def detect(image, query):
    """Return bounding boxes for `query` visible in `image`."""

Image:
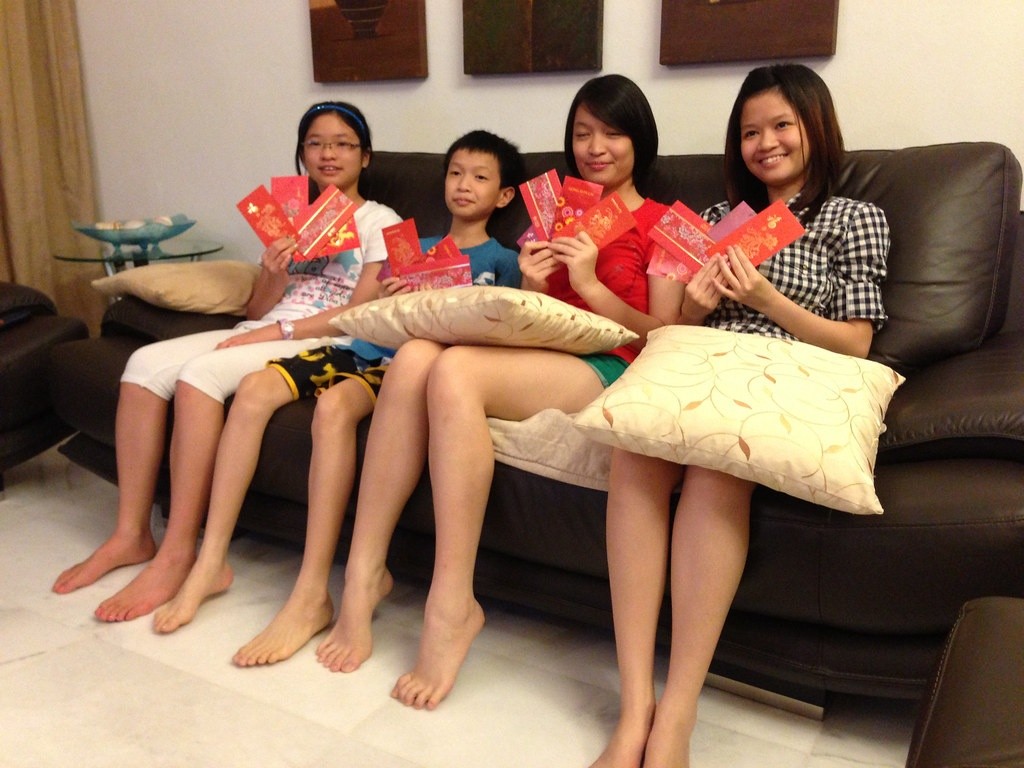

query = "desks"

[51,236,223,302]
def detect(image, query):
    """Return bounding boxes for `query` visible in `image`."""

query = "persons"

[48,100,404,624]
[150,128,523,668]
[590,62,893,767]
[317,76,676,710]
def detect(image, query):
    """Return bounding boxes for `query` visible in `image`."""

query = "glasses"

[300,139,362,154]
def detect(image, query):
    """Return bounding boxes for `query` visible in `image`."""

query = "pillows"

[90,258,265,316]
[328,285,641,354]
[572,324,906,516]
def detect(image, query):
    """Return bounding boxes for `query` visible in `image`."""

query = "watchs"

[277,318,294,341]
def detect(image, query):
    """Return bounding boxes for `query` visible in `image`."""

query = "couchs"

[0,281,90,491]
[903,596,1024,768]
[44,144,1024,704]
